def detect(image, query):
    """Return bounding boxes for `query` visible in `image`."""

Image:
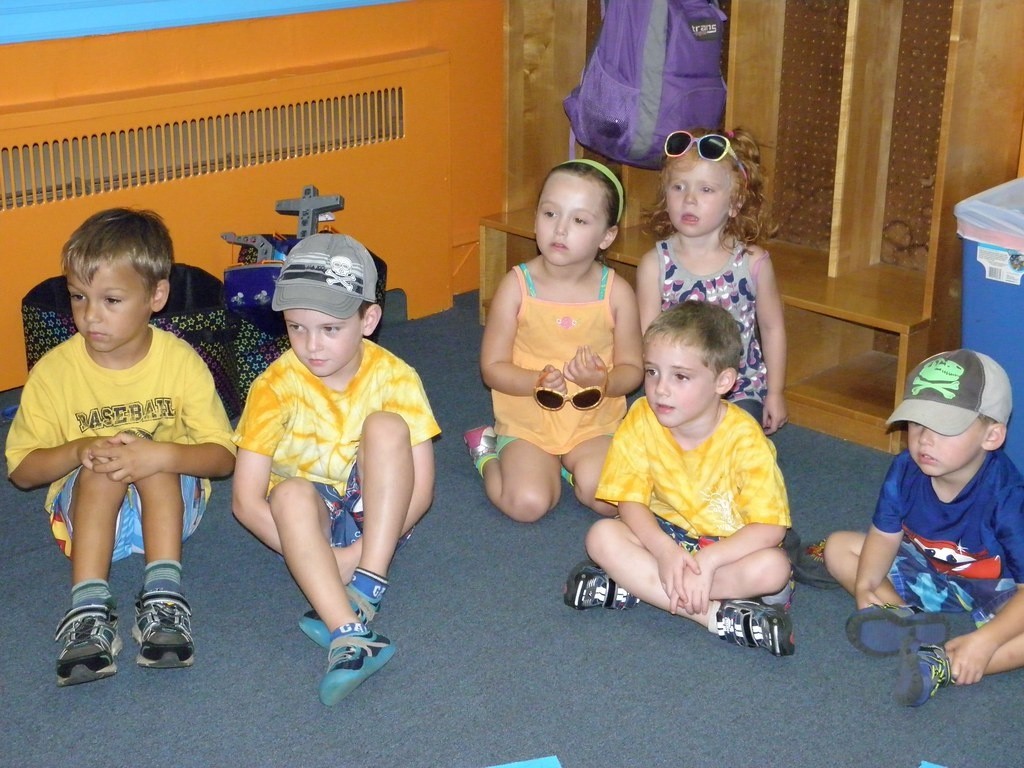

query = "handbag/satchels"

[22,261,240,422]
[222,248,387,414]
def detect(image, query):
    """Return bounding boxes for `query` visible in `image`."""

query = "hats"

[884,348,1013,438]
[270,233,376,320]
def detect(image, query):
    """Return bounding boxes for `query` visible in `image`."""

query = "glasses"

[664,126,749,190]
[533,370,609,411]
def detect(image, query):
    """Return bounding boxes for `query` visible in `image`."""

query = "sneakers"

[320,625,398,703]
[131,590,193,669]
[715,595,795,658]
[298,586,380,649]
[894,641,953,707]
[52,601,121,686]
[463,424,497,459]
[562,559,640,610]
[844,606,951,655]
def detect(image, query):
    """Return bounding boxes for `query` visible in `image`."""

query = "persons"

[4,208,240,686]
[565,300,794,656]
[636,128,790,437]
[463,159,644,522]
[824,349,1023,707]
[233,231,441,708]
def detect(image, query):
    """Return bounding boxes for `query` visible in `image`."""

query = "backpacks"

[562,2,730,171]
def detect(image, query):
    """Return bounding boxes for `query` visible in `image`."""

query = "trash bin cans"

[952,179,1024,477]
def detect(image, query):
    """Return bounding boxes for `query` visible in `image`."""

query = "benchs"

[478,205,930,456]
